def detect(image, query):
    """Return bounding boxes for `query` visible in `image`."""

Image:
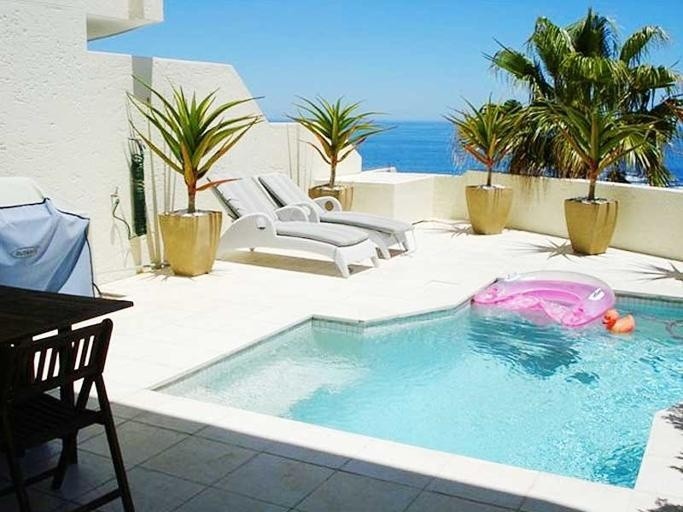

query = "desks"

[1,283,135,465]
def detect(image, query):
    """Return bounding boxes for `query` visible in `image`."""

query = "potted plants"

[123,70,266,277]
[483,5,683,257]
[440,89,522,235]
[285,90,356,210]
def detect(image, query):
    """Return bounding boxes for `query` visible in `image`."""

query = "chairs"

[204,171,380,276]
[256,170,414,259]
[1,319,138,510]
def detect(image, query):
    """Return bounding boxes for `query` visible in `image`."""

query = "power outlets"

[110,194,123,207]
